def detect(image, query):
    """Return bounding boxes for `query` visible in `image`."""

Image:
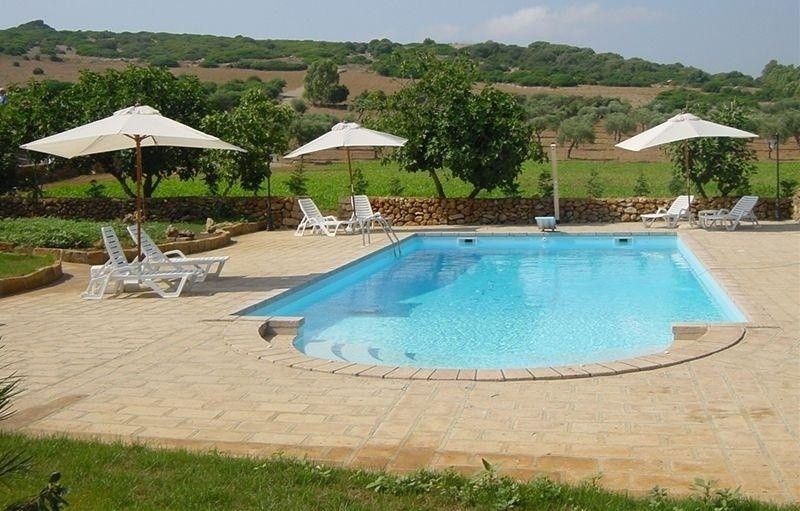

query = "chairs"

[87,222,230,298]
[700,195,760,231]
[639,194,695,228]
[347,193,381,234]
[293,197,354,237]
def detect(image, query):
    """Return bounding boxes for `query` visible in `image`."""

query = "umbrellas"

[281,118,413,227]
[15,102,253,282]
[614,112,761,225]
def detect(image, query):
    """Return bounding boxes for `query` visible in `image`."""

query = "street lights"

[769,132,782,222]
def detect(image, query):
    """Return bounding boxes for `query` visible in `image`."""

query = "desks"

[698,209,719,228]
